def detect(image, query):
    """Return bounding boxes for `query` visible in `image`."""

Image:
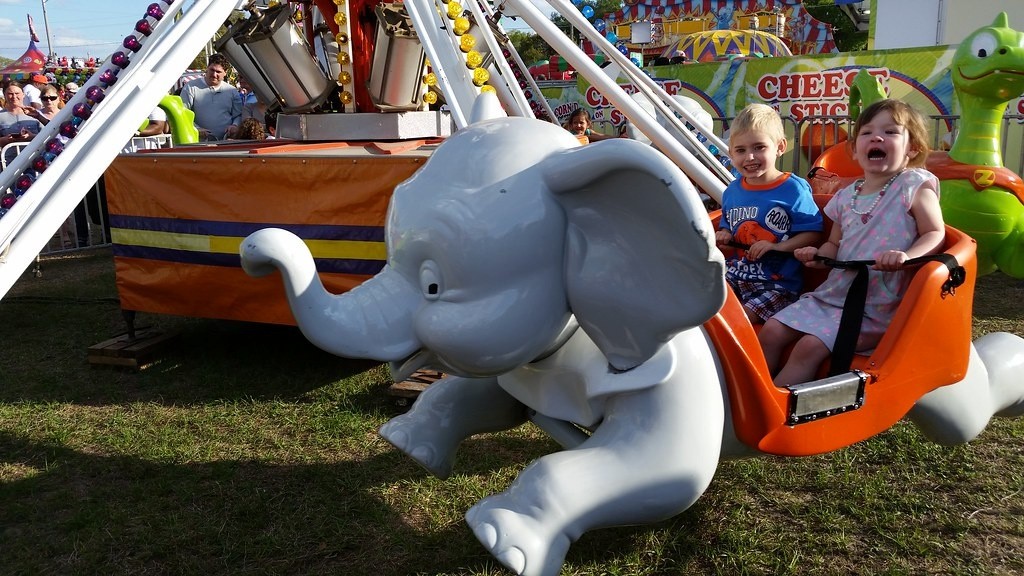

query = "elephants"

[235,113,1024,576]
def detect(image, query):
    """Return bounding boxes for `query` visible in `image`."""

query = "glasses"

[40,96,58,101]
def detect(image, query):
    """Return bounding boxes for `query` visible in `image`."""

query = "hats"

[66,82,79,92]
[33,75,47,83]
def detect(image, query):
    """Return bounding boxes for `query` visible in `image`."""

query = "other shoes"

[70,235,89,249]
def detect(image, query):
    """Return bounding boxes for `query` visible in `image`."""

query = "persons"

[757,98,945,388]
[600,55,612,68]
[37,84,72,248]
[0,79,44,174]
[0,73,184,248]
[715,103,825,323]
[180,54,243,142]
[568,107,628,143]
[235,88,283,139]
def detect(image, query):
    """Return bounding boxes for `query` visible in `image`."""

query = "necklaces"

[850,168,908,224]
